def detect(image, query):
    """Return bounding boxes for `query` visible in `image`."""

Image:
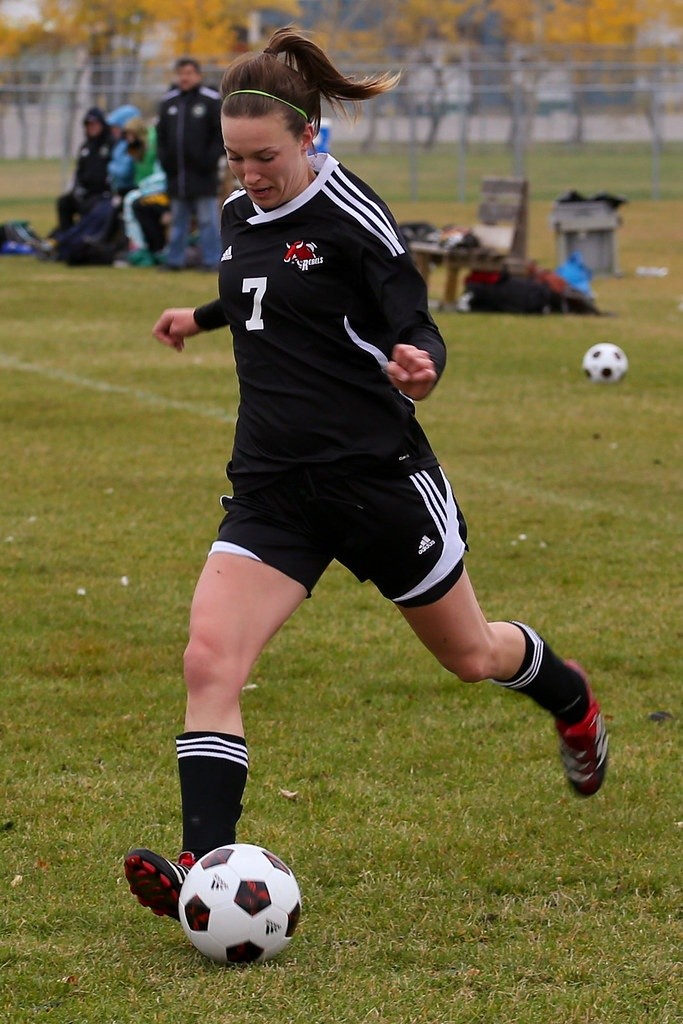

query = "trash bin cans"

[552,201,623,281]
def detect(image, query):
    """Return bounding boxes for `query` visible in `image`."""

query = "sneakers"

[552,658,606,798]
[122,845,196,921]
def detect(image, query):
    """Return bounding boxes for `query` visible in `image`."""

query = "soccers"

[179,844,301,965]
[582,342,628,384]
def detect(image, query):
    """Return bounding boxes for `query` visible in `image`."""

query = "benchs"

[407,234,511,315]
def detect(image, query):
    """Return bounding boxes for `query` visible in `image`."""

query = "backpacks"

[67,191,124,266]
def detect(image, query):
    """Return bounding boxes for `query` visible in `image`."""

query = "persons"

[118,136,161,187]
[37,104,141,259]
[152,56,228,272]
[47,105,116,246]
[121,27,614,931]
[128,192,172,259]
[84,114,151,255]
